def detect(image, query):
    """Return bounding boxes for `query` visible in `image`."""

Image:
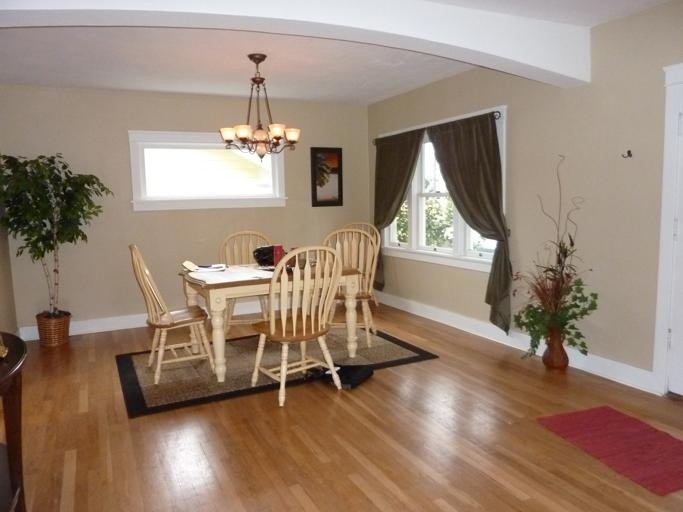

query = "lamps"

[220,54,301,161]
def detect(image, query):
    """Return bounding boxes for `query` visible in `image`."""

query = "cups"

[274,244,283,267]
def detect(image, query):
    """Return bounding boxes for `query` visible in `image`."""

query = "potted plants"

[0,152,114,347]
[509,156,598,370]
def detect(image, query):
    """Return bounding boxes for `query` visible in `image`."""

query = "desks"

[0,332,29,511]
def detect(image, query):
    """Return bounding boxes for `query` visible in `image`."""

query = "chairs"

[320,229,378,358]
[129,244,216,384]
[251,245,343,407]
[219,230,272,335]
[343,222,381,307]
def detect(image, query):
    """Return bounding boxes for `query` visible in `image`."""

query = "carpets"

[115,320,440,418]
[537,404,683,497]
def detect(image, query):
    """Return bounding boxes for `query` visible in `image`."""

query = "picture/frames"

[311,148,343,207]
